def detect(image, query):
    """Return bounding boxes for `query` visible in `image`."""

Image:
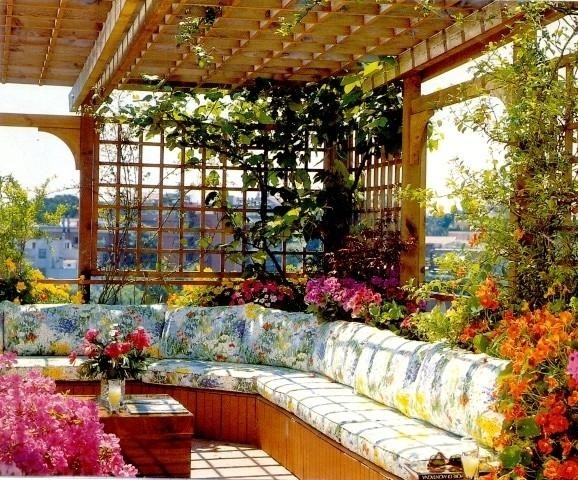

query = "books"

[122,398,190,415]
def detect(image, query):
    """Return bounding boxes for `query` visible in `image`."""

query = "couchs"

[0,298,518,479]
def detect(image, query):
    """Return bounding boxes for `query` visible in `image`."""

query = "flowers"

[64,324,153,383]
[0,353,140,479]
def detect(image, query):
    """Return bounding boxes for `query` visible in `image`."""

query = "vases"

[98,375,126,410]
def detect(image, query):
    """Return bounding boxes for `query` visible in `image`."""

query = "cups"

[107,378,122,412]
